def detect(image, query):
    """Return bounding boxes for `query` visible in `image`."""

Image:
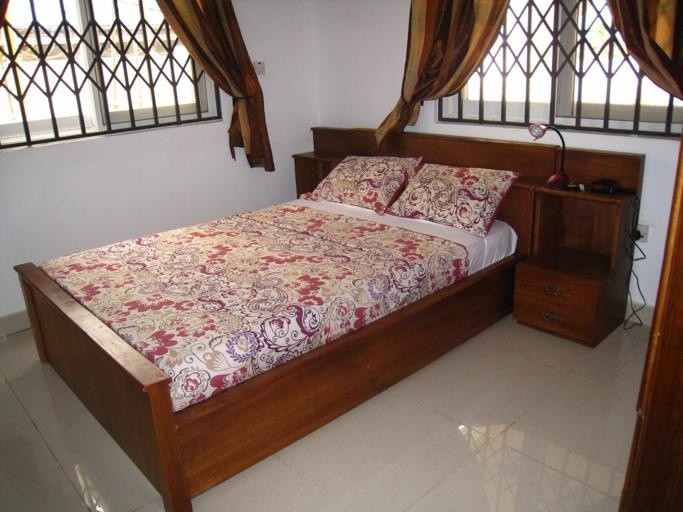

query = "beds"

[13,128,560,512]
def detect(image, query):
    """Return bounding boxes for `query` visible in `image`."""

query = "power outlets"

[636,224,649,243]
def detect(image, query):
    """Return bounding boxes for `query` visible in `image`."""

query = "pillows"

[302,156,423,216]
[384,162,524,238]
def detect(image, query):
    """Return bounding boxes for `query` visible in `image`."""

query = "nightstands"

[513,147,646,348]
[291,126,367,199]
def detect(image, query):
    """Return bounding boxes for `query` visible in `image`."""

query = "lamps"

[528,123,570,189]
